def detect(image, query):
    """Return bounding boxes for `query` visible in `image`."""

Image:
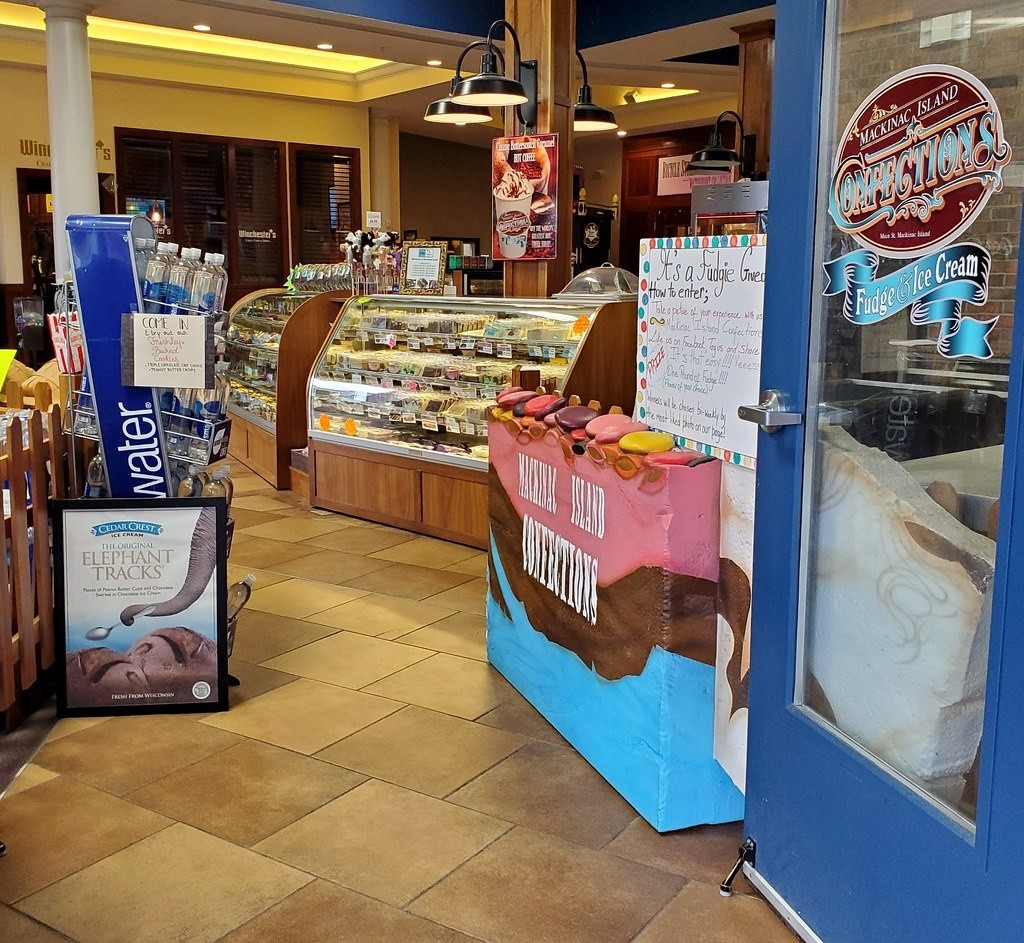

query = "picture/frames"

[402,229,417,241]
[398,240,448,295]
[46,493,229,717]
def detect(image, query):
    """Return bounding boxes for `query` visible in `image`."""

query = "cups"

[494,183,534,259]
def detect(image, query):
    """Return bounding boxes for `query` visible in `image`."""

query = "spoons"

[84,605,156,640]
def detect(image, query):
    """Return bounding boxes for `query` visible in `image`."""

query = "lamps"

[422,40,506,125]
[690,111,759,177]
[573,47,618,131]
[623,87,641,104]
[684,162,732,176]
[449,20,541,138]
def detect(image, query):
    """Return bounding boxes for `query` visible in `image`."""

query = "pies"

[495,138,550,192]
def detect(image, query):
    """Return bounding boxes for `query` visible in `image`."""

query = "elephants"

[119,507,217,628]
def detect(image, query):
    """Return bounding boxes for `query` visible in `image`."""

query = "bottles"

[73,238,233,523]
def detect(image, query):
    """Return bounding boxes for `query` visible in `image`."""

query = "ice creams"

[494,169,533,200]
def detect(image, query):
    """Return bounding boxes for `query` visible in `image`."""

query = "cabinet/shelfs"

[223,287,348,492]
[60,216,243,688]
[308,292,635,552]
